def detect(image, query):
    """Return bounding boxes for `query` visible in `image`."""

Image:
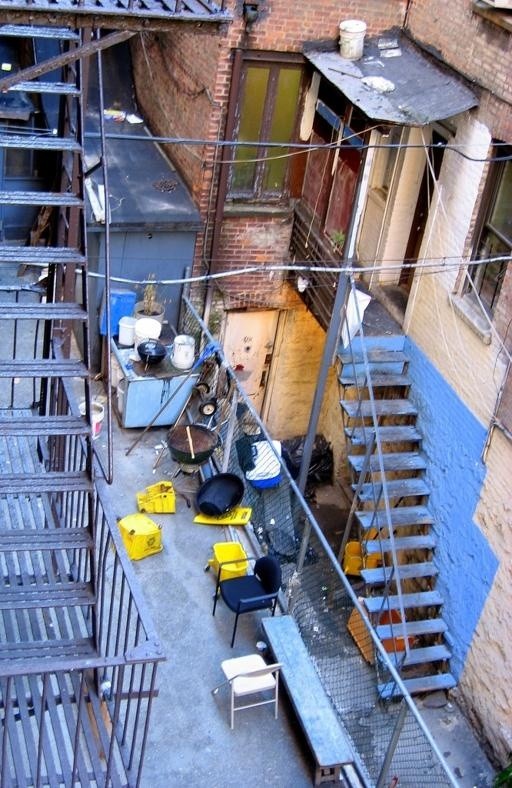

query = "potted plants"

[134,272,165,326]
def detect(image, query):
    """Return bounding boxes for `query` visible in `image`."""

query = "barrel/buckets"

[134,302,166,324]
[172,335,195,369]
[118,316,137,346]
[134,318,162,352]
[344,541,366,576]
[79,402,104,440]
[244,438,283,489]
[339,20,366,59]
[98,287,137,338]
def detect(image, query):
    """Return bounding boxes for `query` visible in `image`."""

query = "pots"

[137,339,166,366]
[196,473,245,516]
[199,400,218,416]
[166,425,219,464]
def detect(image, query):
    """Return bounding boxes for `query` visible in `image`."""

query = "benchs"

[253,614,354,787]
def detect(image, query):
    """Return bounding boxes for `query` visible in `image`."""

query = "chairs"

[212,555,283,730]
